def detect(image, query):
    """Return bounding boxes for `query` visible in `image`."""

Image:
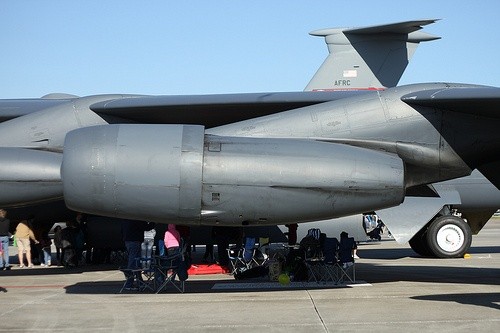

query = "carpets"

[168,263,230,275]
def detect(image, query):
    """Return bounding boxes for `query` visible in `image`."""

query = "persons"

[164,223,180,279]
[0,209,12,270]
[53,213,88,266]
[284,224,357,280]
[121,221,156,290]
[14,222,40,268]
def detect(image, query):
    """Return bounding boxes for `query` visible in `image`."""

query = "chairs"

[118,240,187,294]
[226,237,357,286]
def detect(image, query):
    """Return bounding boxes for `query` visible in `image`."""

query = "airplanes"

[0,18,499,259]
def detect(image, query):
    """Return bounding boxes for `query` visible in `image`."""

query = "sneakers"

[28,264,34,267]
[20,264,27,268]
